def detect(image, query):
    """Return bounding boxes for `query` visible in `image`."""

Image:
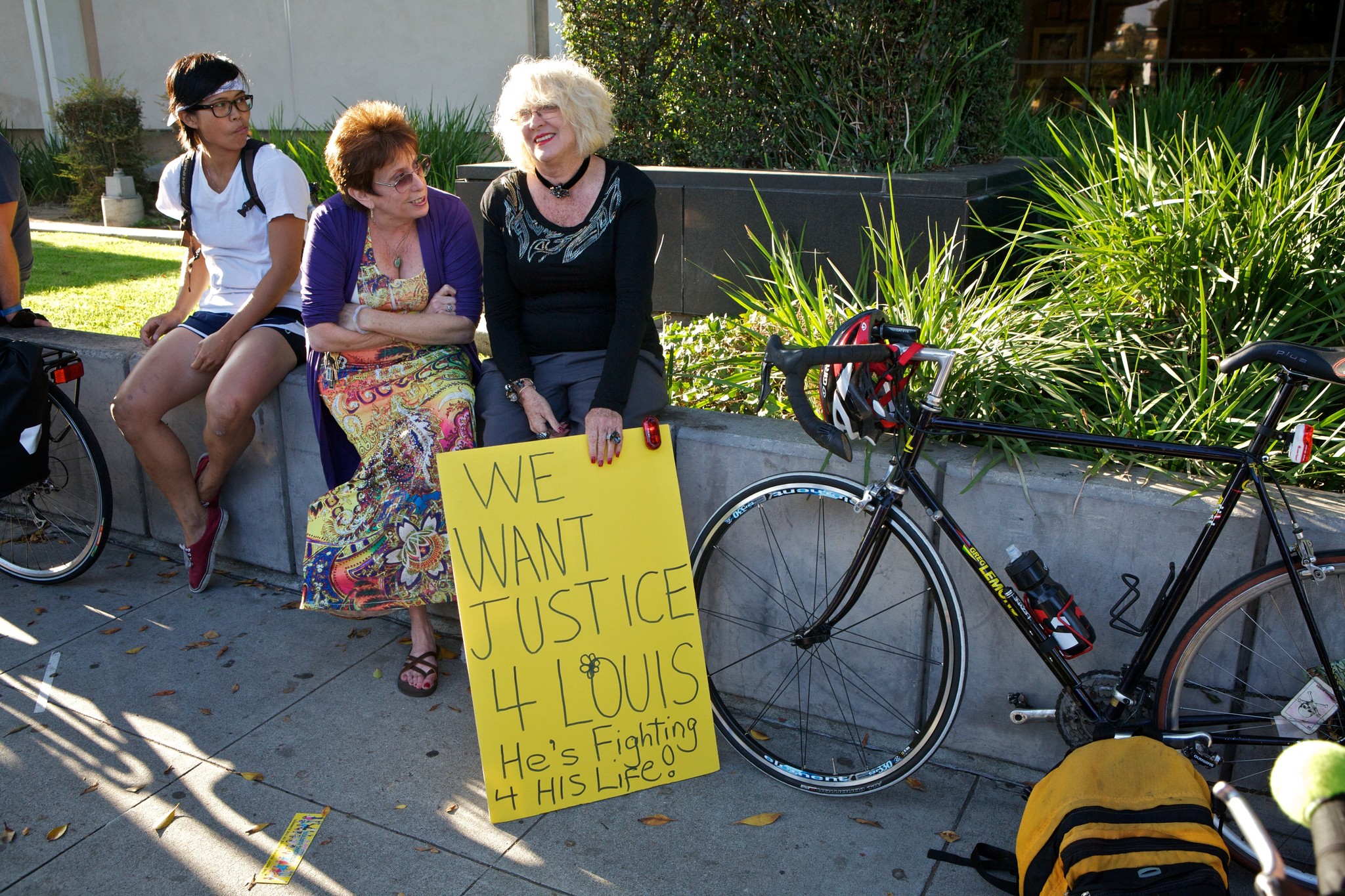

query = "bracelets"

[0,302,23,317]
[353,305,374,335]
[504,378,535,400]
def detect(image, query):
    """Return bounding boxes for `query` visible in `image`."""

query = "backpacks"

[927,735,1231,896]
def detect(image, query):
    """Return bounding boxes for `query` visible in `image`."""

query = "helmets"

[819,309,910,446]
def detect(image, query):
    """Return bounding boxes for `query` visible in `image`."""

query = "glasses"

[510,104,559,127]
[192,95,253,118]
[370,152,432,193]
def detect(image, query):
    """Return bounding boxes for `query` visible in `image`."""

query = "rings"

[606,431,622,444]
[534,432,552,439]
[447,304,452,312]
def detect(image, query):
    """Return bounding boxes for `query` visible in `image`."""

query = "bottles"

[1004,546,1098,654]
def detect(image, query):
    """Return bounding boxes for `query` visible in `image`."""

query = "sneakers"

[194,453,221,508]
[179,507,228,593]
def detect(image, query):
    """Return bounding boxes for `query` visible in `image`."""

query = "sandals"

[398,643,440,697]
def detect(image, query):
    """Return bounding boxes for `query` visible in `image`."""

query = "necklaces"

[369,217,419,267]
[202,154,221,191]
[531,154,591,198]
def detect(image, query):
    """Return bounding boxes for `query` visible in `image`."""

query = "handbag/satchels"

[0,337,51,498]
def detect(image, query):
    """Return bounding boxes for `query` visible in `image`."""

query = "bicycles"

[0,335,111,587]
[687,318,1345,895]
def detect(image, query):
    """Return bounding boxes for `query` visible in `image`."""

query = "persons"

[110,52,311,593]
[299,100,483,698]
[0,129,53,330]
[461,55,666,670]
